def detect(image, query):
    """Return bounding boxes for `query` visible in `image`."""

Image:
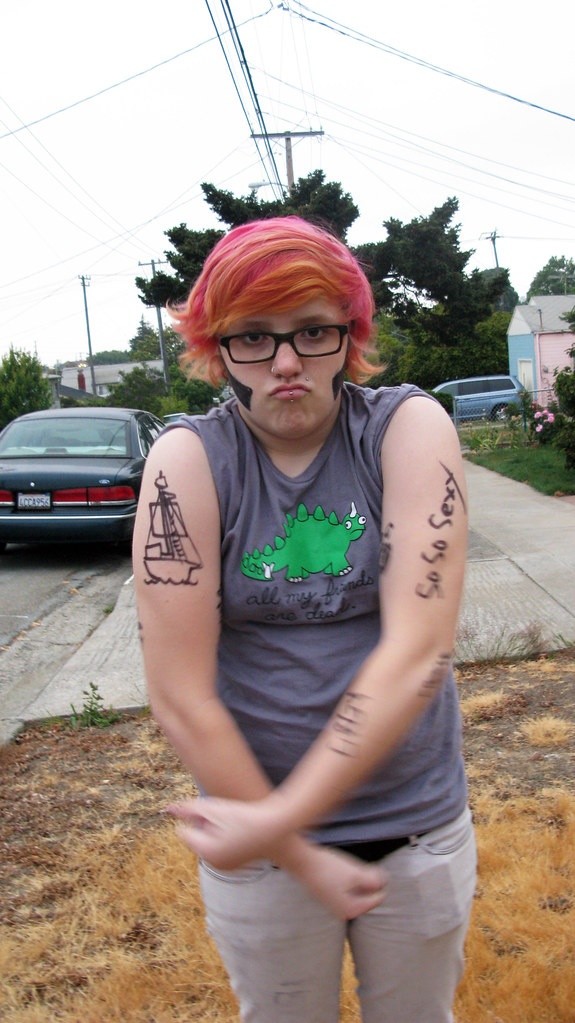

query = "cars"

[0,406,167,553]
[163,412,189,426]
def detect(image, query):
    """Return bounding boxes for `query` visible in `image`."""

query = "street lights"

[248,181,297,198]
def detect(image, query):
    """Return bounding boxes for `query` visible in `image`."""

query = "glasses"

[219,325,349,364]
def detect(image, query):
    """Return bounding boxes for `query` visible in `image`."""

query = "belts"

[336,832,432,861]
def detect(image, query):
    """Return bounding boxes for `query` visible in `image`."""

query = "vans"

[431,375,531,423]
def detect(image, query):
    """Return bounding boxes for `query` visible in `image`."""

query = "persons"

[134,218,477,1023]
